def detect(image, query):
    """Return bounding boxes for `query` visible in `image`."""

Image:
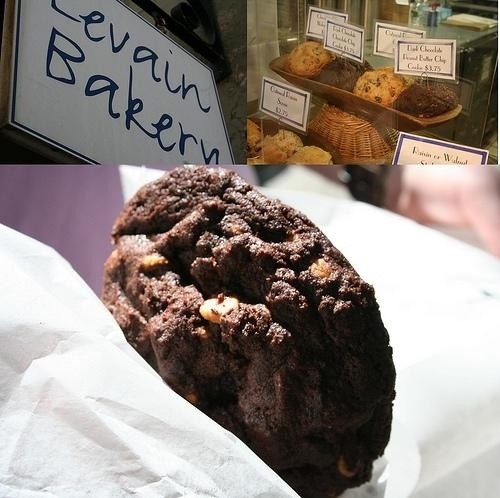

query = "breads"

[258,144,287,165]
[318,60,362,91]
[101,165,397,498]
[284,40,332,79]
[267,129,303,156]
[246,118,263,157]
[353,67,408,107]
[395,82,446,119]
[287,146,333,166]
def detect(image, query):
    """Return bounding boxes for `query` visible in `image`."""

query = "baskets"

[303,97,412,167]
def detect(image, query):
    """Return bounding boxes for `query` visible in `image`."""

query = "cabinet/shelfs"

[248,0,498,165]
